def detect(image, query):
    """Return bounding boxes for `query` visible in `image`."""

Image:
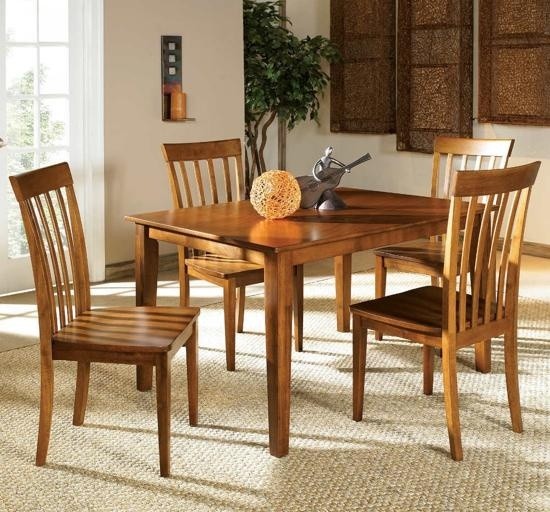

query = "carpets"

[0,266,550,512]
